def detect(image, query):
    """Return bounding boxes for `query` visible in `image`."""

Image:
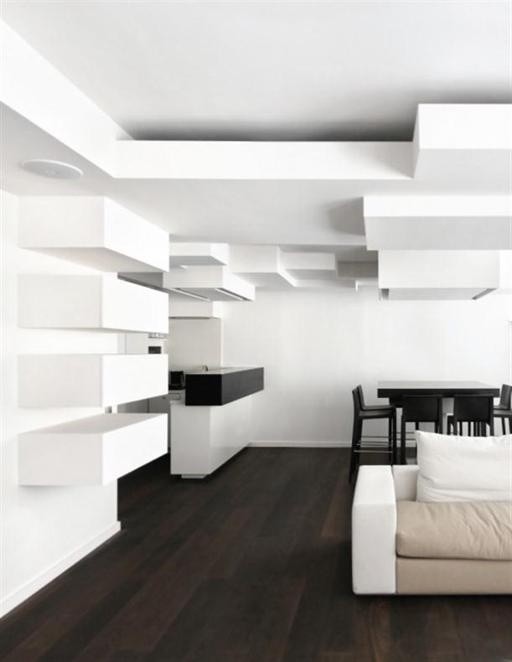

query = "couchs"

[348,427,512,597]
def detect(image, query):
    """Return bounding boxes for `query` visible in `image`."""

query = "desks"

[378,377,503,465]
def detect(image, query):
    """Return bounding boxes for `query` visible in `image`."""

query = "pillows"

[413,427,512,507]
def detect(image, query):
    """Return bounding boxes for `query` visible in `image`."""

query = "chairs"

[443,391,495,437]
[387,397,422,463]
[398,391,446,466]
[355,383,396,465]
[348,386,402,488]
[492,383,508,431]
[493,382,512,435]
